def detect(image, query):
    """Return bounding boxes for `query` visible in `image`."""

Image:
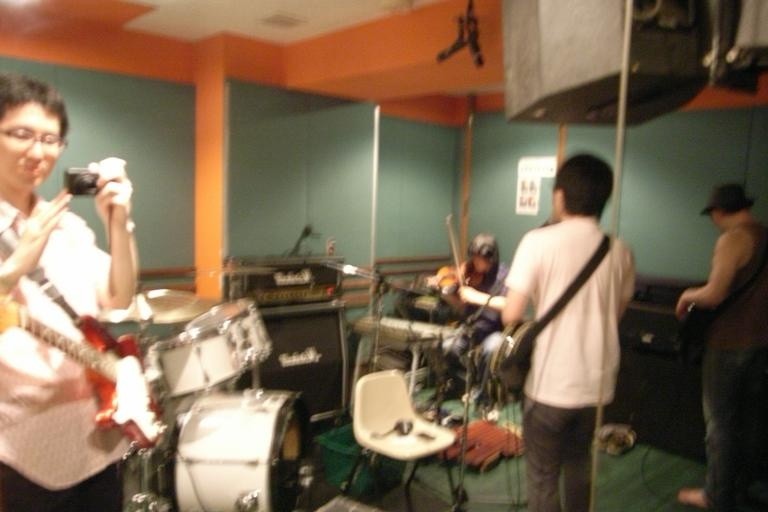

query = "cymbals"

[329,260,433,297]
[129,289,219,321]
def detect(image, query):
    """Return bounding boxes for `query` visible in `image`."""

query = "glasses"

[4,126,71,157]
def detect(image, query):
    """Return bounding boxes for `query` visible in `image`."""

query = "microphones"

[292,223,314,254]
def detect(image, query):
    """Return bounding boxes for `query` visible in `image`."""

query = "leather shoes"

[677,486,706,509]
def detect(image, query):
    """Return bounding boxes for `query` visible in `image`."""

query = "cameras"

[62,166,99,195]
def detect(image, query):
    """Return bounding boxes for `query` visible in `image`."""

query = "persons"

[422,232,509,423]
[674,183,768,512]
[0,73,139,512]
[500,152,635,512]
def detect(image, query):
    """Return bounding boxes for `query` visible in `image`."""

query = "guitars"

[0,298,165,449]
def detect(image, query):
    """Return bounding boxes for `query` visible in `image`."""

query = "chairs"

[350,367,460,511]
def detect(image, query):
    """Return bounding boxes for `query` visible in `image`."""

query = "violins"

[427,260,484,295]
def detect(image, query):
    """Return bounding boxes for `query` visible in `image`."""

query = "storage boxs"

[314,423,407,506]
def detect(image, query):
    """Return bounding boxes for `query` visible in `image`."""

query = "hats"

[699,183,755,216]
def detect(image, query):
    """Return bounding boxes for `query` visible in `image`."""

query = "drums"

[187,297,271,369]
[156,324,240,399]
[176,386,316,512]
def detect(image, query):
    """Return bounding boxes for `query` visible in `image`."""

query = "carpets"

[410,385,707,512]
